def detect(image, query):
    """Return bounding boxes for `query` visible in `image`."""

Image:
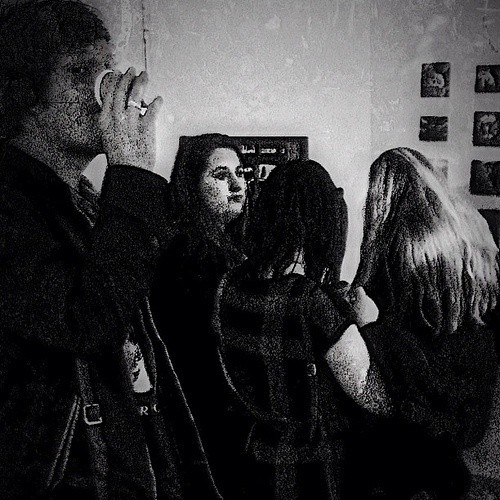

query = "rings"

[125,98,149,117]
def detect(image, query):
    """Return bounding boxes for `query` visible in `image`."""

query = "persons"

[0,0,207,500]
[342,142,497,495]
[151,130,258,353]
[214,153,394,499]
